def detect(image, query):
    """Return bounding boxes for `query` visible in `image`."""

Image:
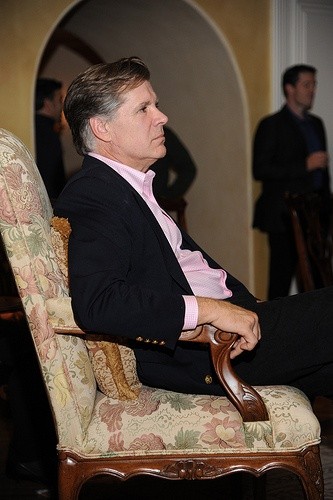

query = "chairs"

[285,195,327,294]
[0,128,330,500]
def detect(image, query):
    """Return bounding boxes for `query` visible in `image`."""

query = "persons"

[0,320,57,482]
[251,64,333,300]
[53,56,333,401]
[148,126,196,214]
[34,77,66,204]
